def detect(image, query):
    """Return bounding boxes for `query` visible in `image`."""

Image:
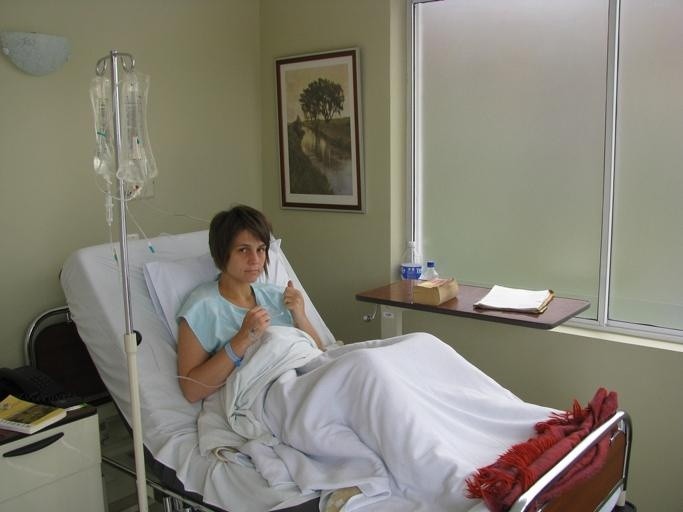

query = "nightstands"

[0,404,107,512]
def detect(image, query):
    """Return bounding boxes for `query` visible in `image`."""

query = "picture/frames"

[273,47,367,214]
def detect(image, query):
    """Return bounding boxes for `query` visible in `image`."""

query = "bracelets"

[225,342,240,362]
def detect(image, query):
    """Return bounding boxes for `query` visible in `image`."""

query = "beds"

[23,227,632,512]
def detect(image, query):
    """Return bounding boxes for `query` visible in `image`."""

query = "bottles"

[419,261,440,282]
[399,241,422,294]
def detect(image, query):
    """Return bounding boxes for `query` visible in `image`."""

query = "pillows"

[141,238,292,346]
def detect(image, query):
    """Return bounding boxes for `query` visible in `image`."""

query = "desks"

[355,281,592,340]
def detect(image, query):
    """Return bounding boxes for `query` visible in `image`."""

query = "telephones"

[0,365,64,404]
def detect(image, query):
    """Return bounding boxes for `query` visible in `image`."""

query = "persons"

[175,204,325,403]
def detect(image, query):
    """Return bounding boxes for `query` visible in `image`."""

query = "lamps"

[0,30,74,76]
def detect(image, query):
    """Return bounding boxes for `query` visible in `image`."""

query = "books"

[0,395,67,435]
[473,284,554,313]
[414,276,458,306]
